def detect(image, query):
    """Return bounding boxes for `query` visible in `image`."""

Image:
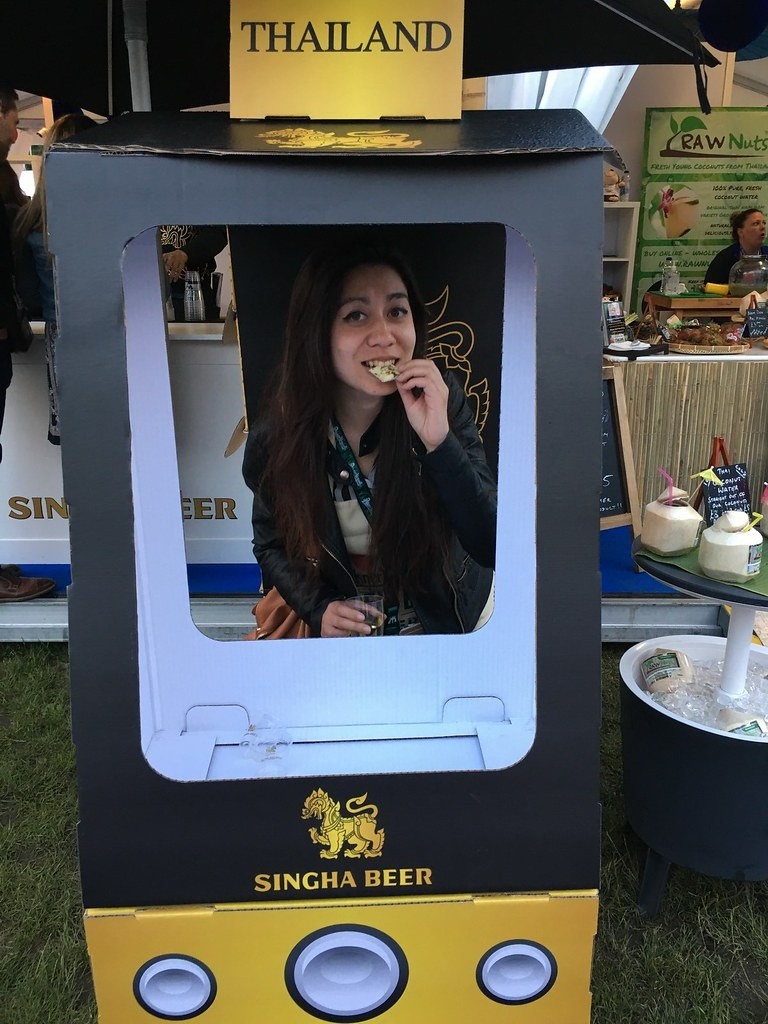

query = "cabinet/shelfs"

[602,200,641,316]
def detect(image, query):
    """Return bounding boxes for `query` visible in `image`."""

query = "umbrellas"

[0,0,722,121]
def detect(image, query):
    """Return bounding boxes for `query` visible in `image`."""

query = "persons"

[704,208,768,286]
[0,81,107,603]
[245,243,497,638]
[160,224,228,322]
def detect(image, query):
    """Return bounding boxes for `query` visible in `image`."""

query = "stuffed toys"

[604,169,625,202]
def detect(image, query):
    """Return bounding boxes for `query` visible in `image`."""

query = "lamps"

[656,0,703,17]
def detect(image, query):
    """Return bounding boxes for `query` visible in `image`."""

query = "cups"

[679,283,685,292]
[183,271,206,321]
[345,594,384,637]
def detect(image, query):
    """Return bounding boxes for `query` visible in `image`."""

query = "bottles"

[639,647,696,693]
[717,707,767,739]
[728,254,768,298]
[640,498,704,558]
[759,501,768,537]
[660,257,680,295]
[697,522,763,583]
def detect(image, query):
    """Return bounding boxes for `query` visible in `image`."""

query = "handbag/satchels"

[247,561,321,640]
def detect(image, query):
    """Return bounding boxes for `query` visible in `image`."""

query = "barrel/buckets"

[617,633,768,882]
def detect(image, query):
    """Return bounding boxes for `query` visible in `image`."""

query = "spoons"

[631,339,649,346]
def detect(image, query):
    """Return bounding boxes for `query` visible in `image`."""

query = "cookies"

[369,364,400,382]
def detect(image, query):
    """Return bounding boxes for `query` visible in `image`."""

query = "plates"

[608,341,651,351]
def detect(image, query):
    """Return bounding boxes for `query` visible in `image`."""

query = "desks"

[649,291,768,329]
[602,338,768,526]
[618,516,768,920]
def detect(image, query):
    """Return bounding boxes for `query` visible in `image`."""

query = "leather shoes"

[1,570,57,602]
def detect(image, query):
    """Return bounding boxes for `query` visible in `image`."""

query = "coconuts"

[639,486,704,556]
[698,510,763,583]
[664,186,700,239]
